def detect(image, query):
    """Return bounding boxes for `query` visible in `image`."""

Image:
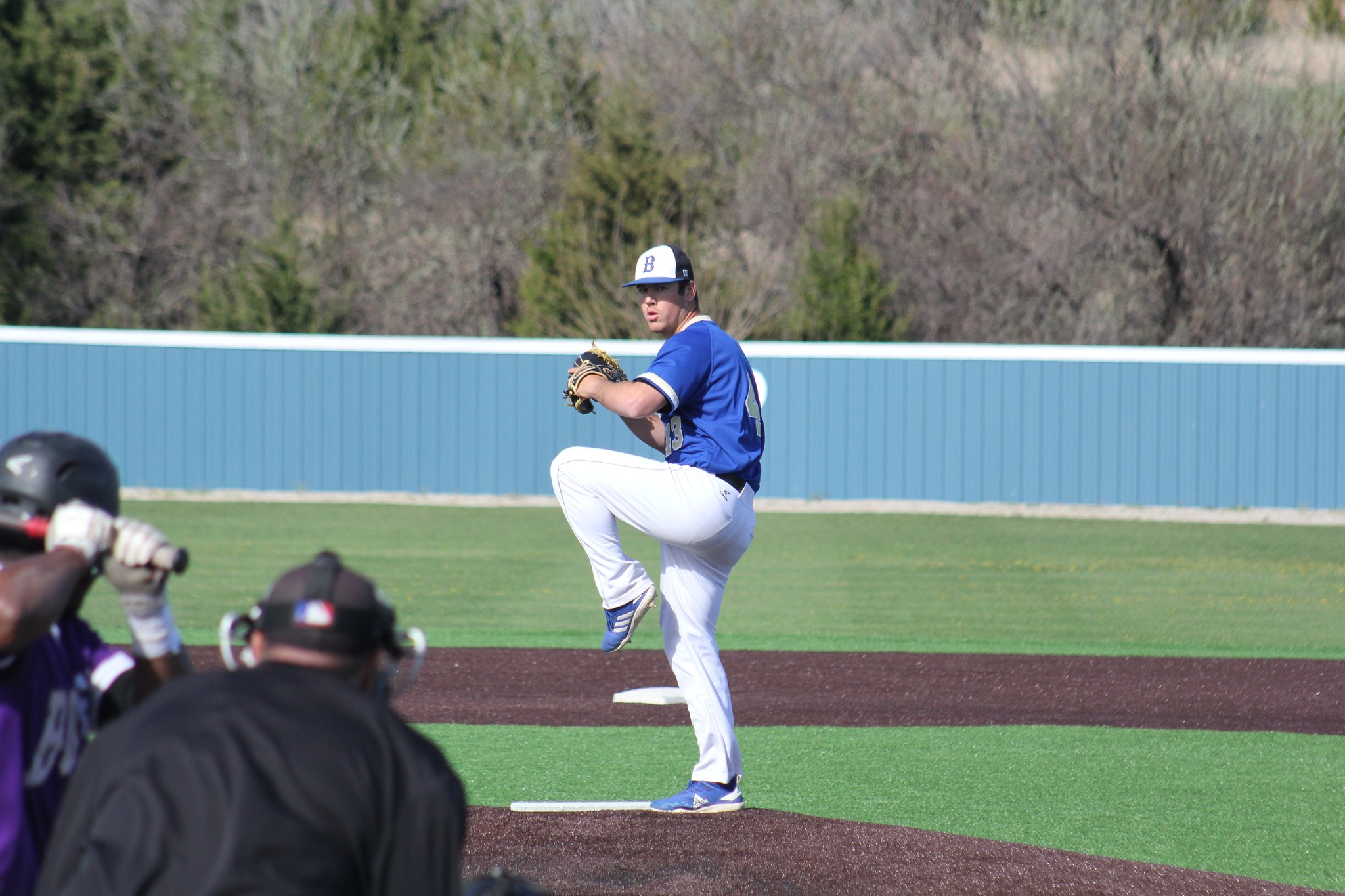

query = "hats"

[253,552,394,656]
[622,245,695,288]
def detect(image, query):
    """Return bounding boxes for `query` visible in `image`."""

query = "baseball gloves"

[567,349,630,414]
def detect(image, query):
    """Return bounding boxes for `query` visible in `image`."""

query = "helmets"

[0,429,120,540]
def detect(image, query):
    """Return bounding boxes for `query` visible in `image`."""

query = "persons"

[0,431,192,896]
[550,243,767,813]
[33,551,548,896]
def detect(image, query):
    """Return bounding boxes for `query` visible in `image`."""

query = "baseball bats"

[0,500,189,574]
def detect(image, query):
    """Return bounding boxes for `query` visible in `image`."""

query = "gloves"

[101,515,183,618]
[43,497,115,570]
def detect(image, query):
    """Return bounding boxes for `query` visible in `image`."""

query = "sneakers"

[650,774,745,813]
[600,585,657,654]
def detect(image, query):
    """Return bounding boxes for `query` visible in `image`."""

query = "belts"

[715,471,746,493]
